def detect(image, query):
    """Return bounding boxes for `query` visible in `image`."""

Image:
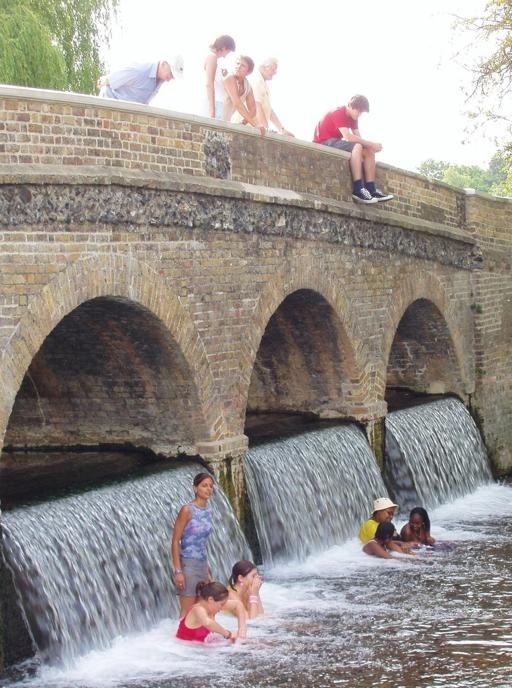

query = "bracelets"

[252,123,256,127]
[224,630,232,639]
[248,596,259,604]
[174,569,182,576]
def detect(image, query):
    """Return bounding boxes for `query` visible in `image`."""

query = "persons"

[171,472,215,618]
[358,497,398,544]
[231,57,295,138]
[175,581,246,643]
[222,55,266,136]
[313,95,394,204]
[400,507,435,545]
[362,521,404,561]
[221,560,264,621]
[184,34,236,119]
[97,52,184,106]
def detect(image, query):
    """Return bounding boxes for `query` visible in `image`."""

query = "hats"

[371,499,398,515]
[163,50,184,81]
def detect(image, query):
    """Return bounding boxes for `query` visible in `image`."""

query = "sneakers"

[352,187,379,203]
[371,191,393,202]
